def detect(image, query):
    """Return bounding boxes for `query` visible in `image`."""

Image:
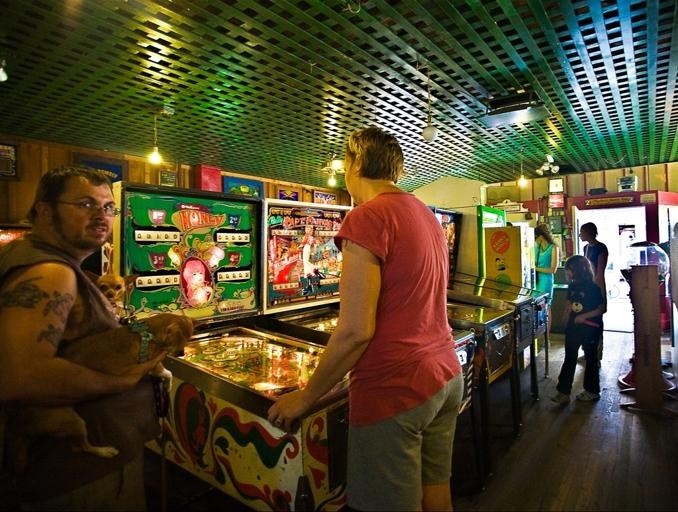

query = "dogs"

[13,312,195,469]
[84,270,139,309]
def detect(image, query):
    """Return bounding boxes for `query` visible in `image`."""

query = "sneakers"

[576,390,601,401]
[551,392,571,404]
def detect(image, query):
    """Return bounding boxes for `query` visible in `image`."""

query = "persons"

[576,223,605,364]
[531,227,558,349]
[3,165,168,507]
[553,256,610,402]
[265,125,465,510]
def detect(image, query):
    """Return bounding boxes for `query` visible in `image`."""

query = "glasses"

[57,201,120,216]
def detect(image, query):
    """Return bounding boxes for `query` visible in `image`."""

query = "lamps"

[322,147,342,188]
[516,144,533,189]
[417,55,440,144]
[145,107,176,165]
